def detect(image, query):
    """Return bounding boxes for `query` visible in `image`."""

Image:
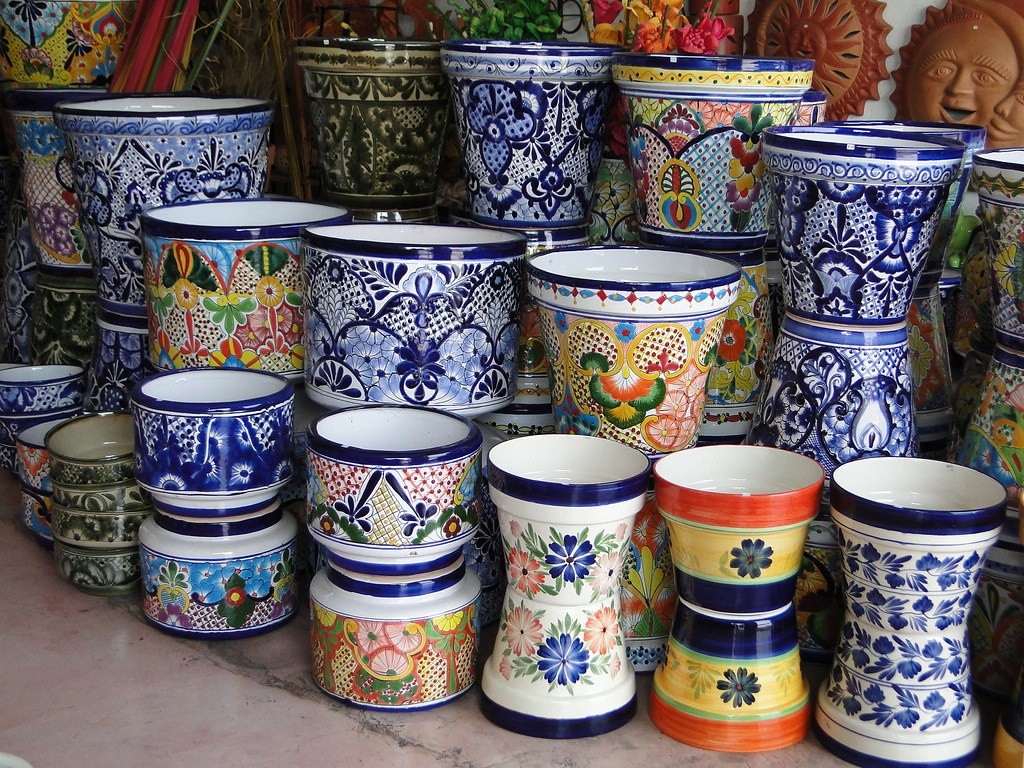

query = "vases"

[1,0,1023,767]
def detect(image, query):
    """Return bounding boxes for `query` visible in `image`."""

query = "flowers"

[585,0,734,158]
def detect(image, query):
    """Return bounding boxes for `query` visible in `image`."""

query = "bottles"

[0,1,1023,767]
